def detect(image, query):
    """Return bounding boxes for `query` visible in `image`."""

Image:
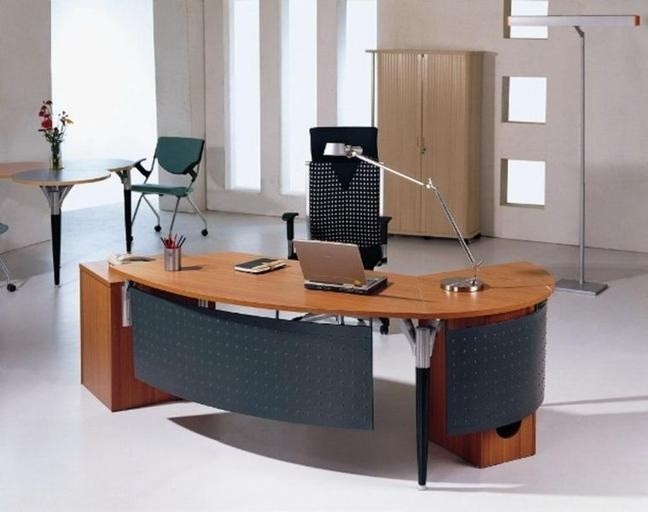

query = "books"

[231,257,285,275]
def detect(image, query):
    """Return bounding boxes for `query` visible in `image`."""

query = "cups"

[163,245,181,272]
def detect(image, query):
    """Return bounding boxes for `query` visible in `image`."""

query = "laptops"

[293,240,387,295]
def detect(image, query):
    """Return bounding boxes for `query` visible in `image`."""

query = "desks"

[77,246,556,490]
[0,156,135,289]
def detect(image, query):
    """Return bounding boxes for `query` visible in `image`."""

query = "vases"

[47,139,64,170]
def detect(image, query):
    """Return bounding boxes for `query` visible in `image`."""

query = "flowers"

[38,97,74,170]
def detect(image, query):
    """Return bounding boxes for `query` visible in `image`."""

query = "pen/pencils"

[159,232,186,248]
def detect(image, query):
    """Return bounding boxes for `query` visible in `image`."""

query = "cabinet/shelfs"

[365,46,484,244]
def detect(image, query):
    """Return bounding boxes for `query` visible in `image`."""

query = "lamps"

[320,141,486,291]
[499,12,641,299]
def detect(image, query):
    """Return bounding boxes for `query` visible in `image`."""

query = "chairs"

[0,222,19,293]
[281,126,393,273]
[128,136,210,247]
